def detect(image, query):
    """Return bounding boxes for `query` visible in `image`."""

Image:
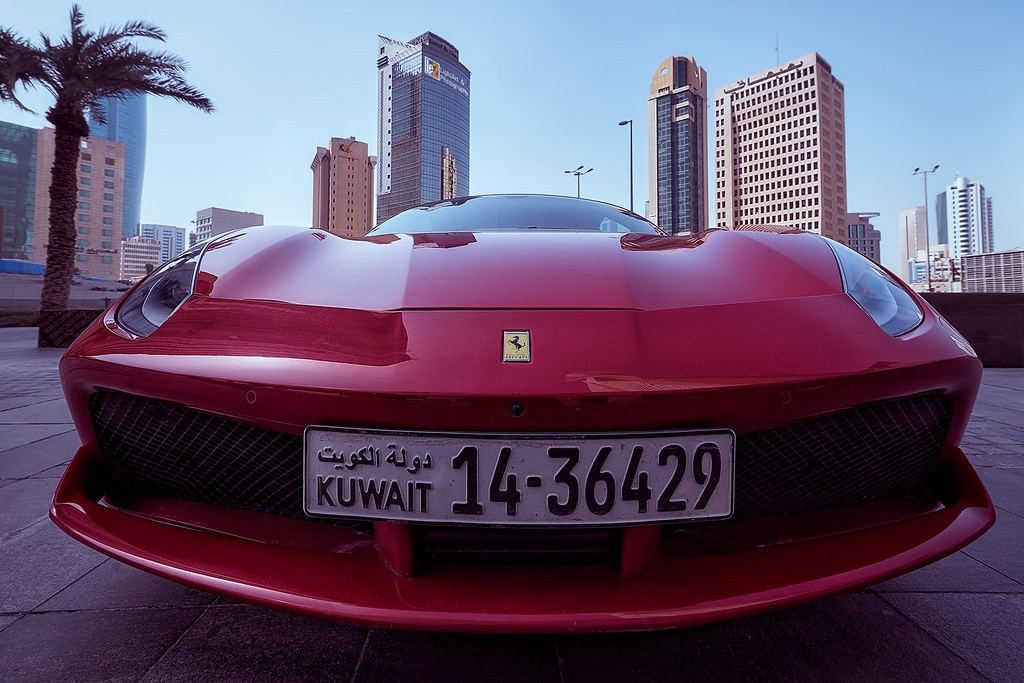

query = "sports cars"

[49,193,997,638]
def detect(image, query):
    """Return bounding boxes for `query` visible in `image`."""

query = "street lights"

[564,166,593,199]
[913,165,939,292]
[619,119,634,211]
